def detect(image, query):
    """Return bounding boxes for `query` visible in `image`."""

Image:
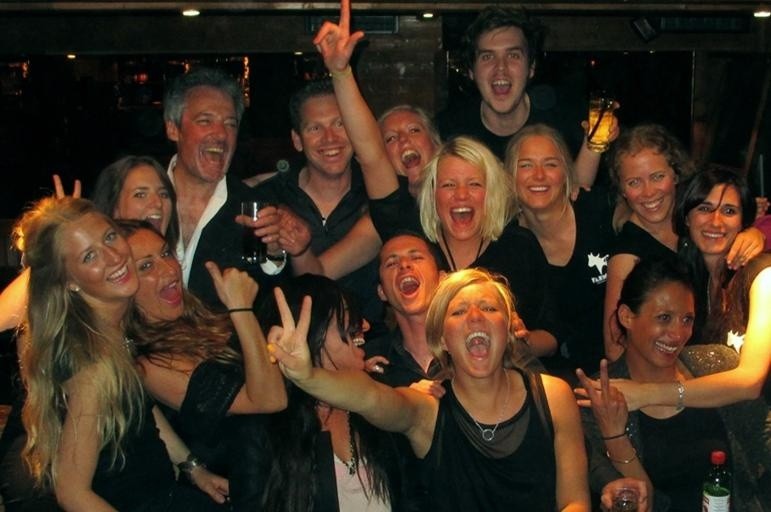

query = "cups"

[585,88,617,154]
[237,200,271,264]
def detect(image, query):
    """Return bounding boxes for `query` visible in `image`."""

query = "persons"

[1,1,771,511]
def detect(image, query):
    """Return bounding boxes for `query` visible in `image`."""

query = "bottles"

[700,452,736,511]
[609,487,644,512]
